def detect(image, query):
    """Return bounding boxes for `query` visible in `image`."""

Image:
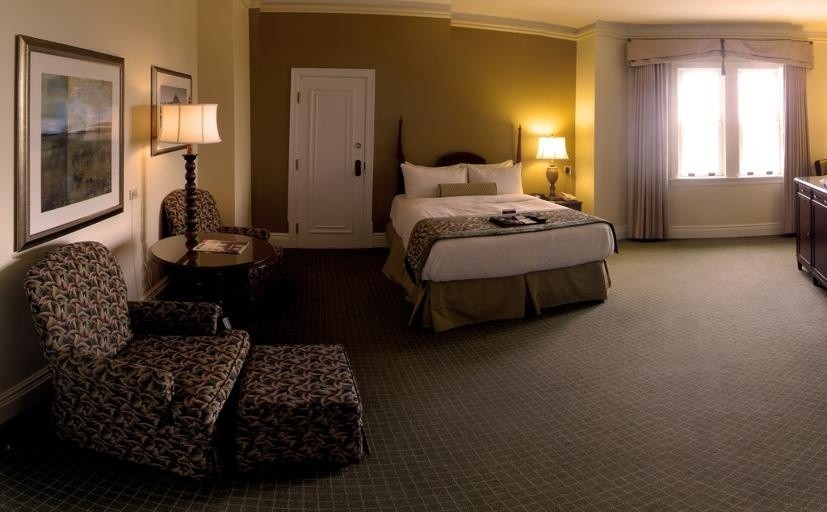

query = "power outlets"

[564,166,572,174]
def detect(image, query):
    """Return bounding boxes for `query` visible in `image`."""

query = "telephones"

[560,192,577,201]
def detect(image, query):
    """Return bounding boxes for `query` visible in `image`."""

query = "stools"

[230,343,365,475]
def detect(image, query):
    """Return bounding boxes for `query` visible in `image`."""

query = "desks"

[794,176,827,291]
[147,233,278,331]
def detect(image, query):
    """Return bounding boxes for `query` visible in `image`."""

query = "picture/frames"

[13,35,123,253]
[150,66,192,157]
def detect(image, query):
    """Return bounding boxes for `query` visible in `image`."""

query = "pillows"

[398,159,523,199]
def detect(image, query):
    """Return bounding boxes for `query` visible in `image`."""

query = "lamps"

[535,137,570,199]
[158,103,222,250]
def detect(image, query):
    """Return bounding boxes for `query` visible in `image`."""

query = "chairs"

[23,240,251,481]
[160,189,273,290]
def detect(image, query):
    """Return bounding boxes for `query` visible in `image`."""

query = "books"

[191,239,249,255]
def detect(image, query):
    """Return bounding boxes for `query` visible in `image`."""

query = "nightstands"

[549,200,582,212]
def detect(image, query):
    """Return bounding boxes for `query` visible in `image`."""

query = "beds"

[385,116,617,332]
